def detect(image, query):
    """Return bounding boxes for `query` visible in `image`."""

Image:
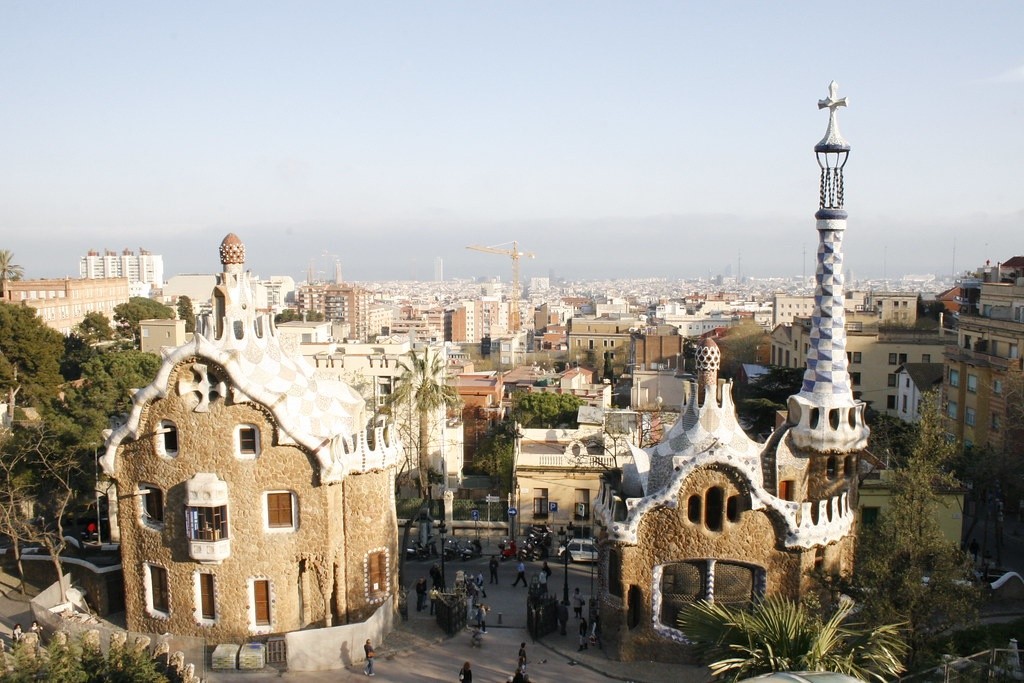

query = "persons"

[577,618,588,651]
[465,570,487,598]
[86,520,96,542]
[538,560,551,593]
[430,562,446,591]
[511,558,527,587]
[506,668,531,683]
[363,639,375,676]
[471,603,488,649]
[459,661,472,683]
[517,642,526,673]
[557,601,568,635]
[428,584,438,615]
[416,575,428,612]
[489,555,499,586]
[573,588,585,619]
[589,616,602,649]
[472,537,482,557]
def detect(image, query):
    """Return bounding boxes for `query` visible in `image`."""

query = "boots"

[584,642,588,650]
[577,645,583,651]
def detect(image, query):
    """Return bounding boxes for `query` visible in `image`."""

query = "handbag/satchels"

[459,670,464,680]
[368,652,374,658]
[517,656,525,663]
[580,601,585,606]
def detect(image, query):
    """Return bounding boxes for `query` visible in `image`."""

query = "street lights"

[437,519,447,590]
[558,521,577,606]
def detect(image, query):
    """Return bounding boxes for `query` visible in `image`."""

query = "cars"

[558,537,599,563]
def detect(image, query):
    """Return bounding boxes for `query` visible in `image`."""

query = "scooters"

[497,537,519,562]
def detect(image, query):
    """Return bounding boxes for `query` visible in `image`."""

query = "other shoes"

[369,673,375,676]
[363,671,367,676]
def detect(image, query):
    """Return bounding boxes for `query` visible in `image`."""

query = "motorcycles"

[443,538,465,561]
[517,533,552,563]
[405,535,440,562]
[461,537,483,561]
